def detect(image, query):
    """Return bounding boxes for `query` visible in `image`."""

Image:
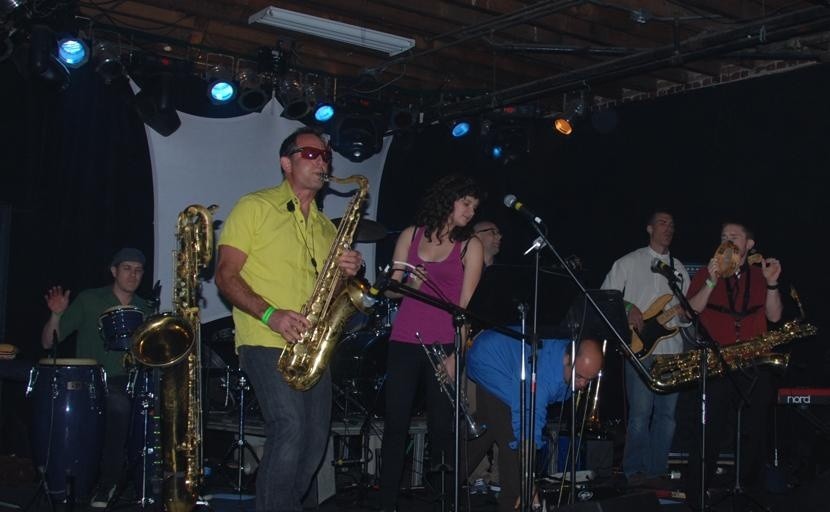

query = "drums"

[25,357,106,505]
[333,332,428,419]
[97,303,146,351]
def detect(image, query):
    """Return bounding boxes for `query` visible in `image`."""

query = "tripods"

[704,389,772,512]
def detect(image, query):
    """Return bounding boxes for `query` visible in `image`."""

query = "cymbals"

[328,217,386,243]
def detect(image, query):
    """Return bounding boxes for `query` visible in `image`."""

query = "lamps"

[556,89,588,135]
[275,74,310,120]
[93,31,126,84]
[51,15,94,68]
[435,99,472,139]
[204,51,238,108]
[305,81,336,125]
[233,63,269,113]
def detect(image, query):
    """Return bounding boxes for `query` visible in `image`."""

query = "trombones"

[556,339,613,512]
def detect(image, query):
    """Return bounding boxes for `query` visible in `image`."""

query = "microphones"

[648,258,682,282]
[504,194,543,224]
[361,267,394,307]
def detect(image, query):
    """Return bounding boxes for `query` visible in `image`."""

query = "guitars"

[630,293,690,358]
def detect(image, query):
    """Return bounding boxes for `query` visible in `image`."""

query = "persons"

[461,224,520,501]
[601,210,690,497]
[466,326,603,511]
[42,247,159,508]
[379,175,482,512]
[685,217,782,512]
[215,128,363,512]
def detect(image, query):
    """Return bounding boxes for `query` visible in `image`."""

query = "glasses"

[287,146,333,163]
[476,228,504,237]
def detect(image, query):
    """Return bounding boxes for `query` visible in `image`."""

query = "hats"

[111,247,146,266]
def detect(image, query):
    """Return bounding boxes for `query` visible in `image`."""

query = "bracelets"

[767,283,778,290]
[261,306,276,324]
[705,279,717,288]
[625,302,634,312]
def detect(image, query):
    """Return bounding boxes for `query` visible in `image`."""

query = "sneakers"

[91,482,116,508]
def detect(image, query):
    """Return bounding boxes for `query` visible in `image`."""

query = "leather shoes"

[628,471,648,487]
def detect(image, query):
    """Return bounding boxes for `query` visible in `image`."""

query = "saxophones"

[277,162,376,395]
[130,198,225,507]
[648,287,814,394]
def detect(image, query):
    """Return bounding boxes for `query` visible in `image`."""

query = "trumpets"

[410,324,492,445]
